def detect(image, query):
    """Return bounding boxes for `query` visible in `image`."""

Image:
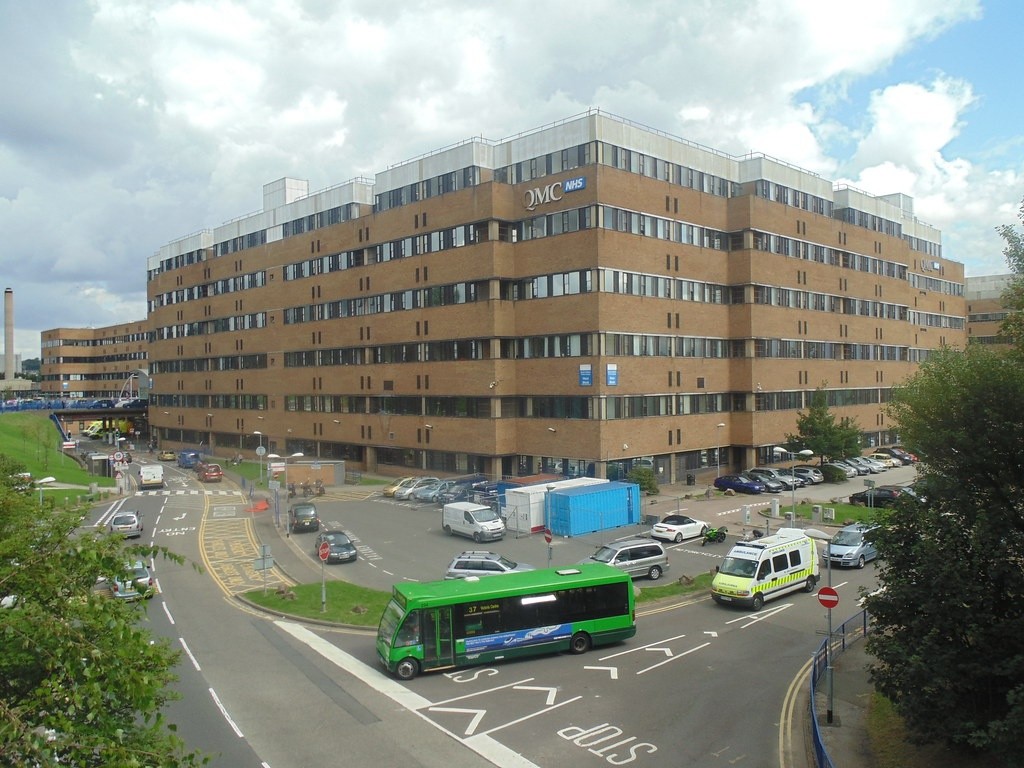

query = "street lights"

[544,483,556,569]
[267,452,304,502]
[773,447,814,524]
[253,430,263,485]
[803,527,835,724]
[716,423,727,477]
[38,477,56,525]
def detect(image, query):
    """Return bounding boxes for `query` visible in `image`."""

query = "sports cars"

[651,513,712,543]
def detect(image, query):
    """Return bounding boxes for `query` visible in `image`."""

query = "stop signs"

[318,541,330,561]
[544,529,552,543]
[817,587,839,608]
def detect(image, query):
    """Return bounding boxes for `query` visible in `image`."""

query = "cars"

[578,538,670,584]
[197,463,223,483]
[108,560,154,603]
[109,510,144,539]
[823,520,884,569]
[0,390,150,409]
[713,445,924,495]
[384,475,489,508]
[316,530,357,564]
[68,422,121,440]
[287,502,319,533]
[158,450,175,462]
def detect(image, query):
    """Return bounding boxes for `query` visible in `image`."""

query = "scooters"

[286,478,326,499]
[740,528,764,542]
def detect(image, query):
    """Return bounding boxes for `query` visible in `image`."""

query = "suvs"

[445,550,535,581]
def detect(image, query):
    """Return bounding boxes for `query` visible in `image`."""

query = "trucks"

[472,473,571,516]
[137,465,164,489]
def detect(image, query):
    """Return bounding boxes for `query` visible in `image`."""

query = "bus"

[377,563,636,680]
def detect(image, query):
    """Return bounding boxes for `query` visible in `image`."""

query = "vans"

[442,502,507,543]
[178,449,202,469]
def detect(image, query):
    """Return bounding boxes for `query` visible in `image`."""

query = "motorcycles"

[701,524,728,546]
[711,527,822,611]
